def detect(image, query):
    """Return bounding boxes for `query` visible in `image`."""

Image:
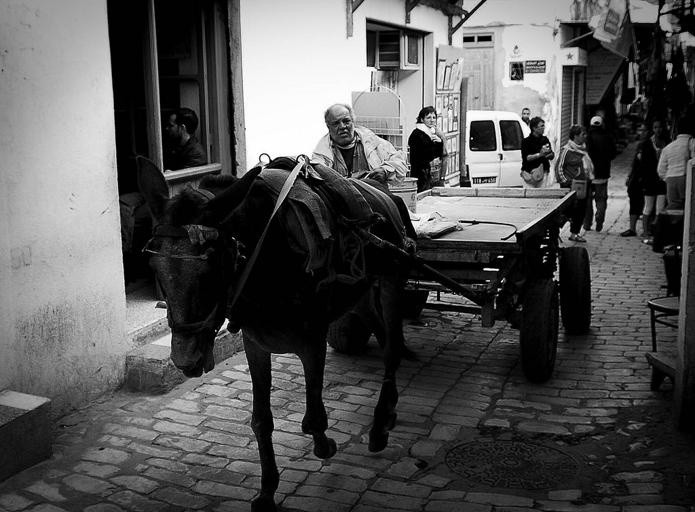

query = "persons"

[520,103,695,246]
[408,106,450,192]
[311,104,408,191]
[118,105,208,276]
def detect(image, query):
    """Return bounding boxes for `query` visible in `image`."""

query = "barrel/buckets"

[389,176,417,215]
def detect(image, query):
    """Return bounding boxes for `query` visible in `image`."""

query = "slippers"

[569,234,587,243]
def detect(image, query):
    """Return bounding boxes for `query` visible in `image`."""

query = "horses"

[132,152,423,512]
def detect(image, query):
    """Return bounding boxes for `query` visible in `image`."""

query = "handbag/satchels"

[519,161,551,186]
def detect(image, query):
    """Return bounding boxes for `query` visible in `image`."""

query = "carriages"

[119,152,594,510]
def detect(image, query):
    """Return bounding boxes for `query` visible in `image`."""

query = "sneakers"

[621,229,636,237]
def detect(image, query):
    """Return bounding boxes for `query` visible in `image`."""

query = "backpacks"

[571,177,590,200]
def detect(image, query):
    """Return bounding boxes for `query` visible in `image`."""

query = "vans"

[459,108,535,188]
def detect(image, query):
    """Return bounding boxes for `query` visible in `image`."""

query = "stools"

[647,295,679,355]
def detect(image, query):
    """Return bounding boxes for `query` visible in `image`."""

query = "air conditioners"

[377,28,420,71]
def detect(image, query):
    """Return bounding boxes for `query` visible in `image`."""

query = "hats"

[589,115,604,126]
